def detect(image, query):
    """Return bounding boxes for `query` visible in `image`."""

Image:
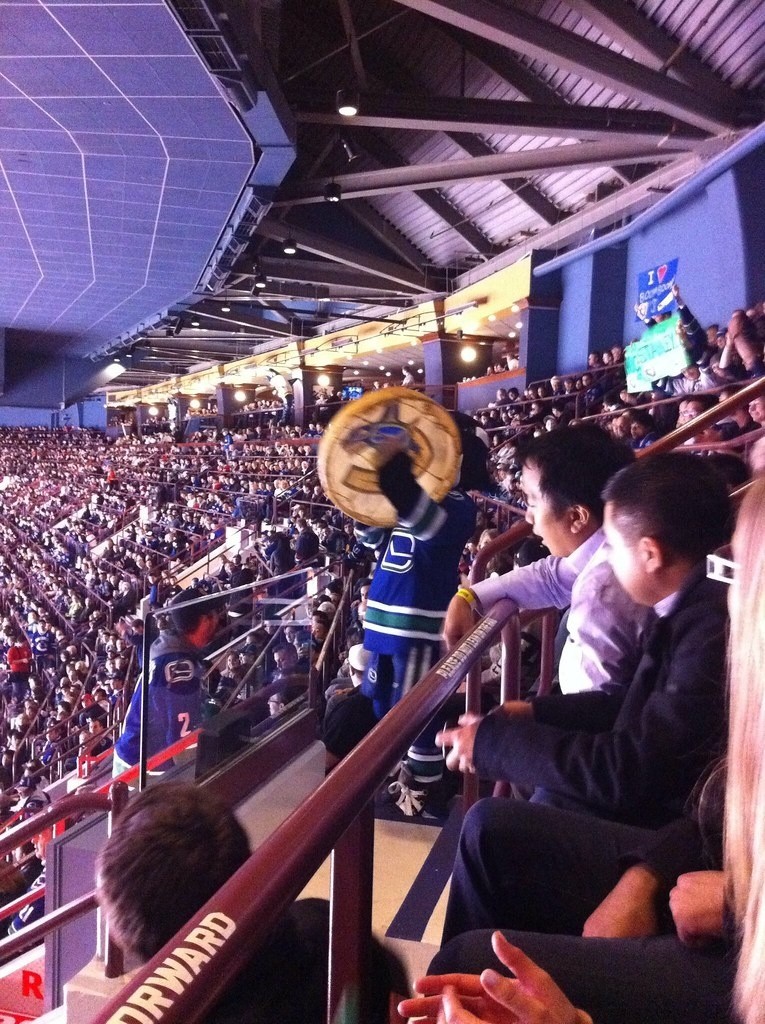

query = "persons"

[0,288,765,1024]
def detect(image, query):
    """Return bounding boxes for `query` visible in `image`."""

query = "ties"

[644,607,657,631]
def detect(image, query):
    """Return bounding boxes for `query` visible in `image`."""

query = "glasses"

[682,409,701,417]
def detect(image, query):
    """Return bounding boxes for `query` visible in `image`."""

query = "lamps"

[342,136,361,163]
[113,354,121,362]
[254,265,267,289]
[190,310,200,326]
[166,328,174,338]
[324,183,342,203]
[337,88,361,117]
[457,330,463,339]
[282,230,297,255]
[125,348,135,358]
[221,288,231,313]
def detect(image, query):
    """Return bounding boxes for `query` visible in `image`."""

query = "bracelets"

[456,588,477,610]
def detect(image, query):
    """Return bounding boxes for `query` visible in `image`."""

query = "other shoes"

[375,763,442,818]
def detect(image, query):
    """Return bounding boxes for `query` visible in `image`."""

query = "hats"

[349,643,373,672]
[542,415,556,423]
[497,457,509,470]
